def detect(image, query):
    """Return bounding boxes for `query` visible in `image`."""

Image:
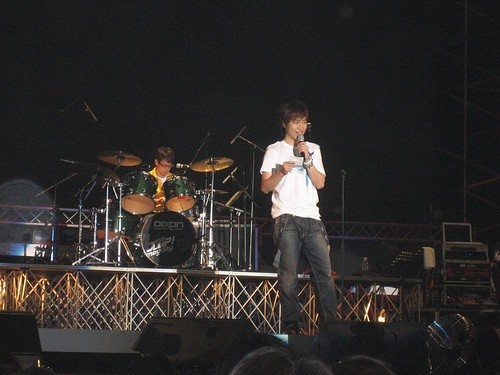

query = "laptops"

[443,222,483,245]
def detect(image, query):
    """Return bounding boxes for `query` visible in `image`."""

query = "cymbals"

[195,188,229,195]
[97,150,143,167]
[190,156,235,173]
[79,164,120,186]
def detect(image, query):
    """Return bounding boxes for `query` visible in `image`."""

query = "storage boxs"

[435,223,490,305]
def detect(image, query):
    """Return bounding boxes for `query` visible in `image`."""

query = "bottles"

[361,257,369,277]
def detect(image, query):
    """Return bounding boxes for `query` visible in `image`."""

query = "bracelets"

[304,159,313,169]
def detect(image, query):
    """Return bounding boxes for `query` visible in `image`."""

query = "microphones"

[231,126,246,144]
[83,100,98,121]
[223,167,238,183]
[176,164,190,168]
[297,134,305,157]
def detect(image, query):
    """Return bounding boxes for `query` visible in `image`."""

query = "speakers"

[0,310,42,375]
[132,319,248,360]
[306,319,429,370]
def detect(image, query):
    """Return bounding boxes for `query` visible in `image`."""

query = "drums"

[129,211,197,268]
[113,167,159,216]
[161,175,199,212]
[97,198,138,245]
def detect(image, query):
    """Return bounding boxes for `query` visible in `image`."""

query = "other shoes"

[285,323,300,336]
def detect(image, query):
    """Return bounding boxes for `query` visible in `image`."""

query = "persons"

[133,151,178,212]
[229,346,393,375]
[259,100,337,333]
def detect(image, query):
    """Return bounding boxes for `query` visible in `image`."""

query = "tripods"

[27,159,137,266]
